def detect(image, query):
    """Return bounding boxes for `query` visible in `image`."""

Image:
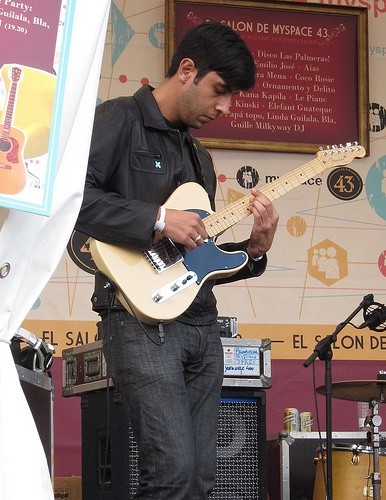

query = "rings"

[195,234,201,242]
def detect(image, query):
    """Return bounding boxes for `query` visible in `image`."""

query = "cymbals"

[315,378,385,403]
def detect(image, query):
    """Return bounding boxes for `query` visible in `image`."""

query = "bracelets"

[154,206,167,233]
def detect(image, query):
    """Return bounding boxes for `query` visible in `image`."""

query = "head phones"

[10,327,55,377]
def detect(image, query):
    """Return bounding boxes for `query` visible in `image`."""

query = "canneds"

[283,408,313,431]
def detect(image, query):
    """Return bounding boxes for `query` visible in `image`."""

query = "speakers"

[81,387,268,500]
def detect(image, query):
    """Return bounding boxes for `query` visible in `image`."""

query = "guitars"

[90,139,367,326]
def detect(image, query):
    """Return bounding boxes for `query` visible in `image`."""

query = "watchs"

[247,242,264,262]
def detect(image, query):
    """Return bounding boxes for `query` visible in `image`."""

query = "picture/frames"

[165,0,371,156]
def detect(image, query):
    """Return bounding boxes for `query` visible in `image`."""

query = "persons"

[72,23,281,500]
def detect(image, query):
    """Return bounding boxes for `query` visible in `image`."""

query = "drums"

[310,441,386,500]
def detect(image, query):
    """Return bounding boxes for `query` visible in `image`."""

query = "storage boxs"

[278,430,386,500]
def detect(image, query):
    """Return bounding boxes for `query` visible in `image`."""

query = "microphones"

[359,306,386,329]
